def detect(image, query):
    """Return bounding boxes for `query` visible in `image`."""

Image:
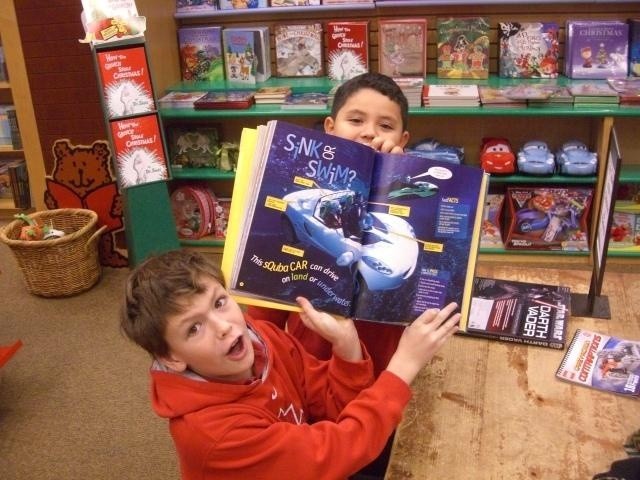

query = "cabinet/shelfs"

[152,0,640,261]
[0,35,35,214]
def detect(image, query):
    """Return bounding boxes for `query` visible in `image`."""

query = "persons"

[245,75,413,479]
[114,245,464,480]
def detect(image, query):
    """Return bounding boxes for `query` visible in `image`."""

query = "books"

[166,0,639,109]
[460,263,573,351]
[552,325,640,405]
[214,119,493,344]
[0,49,33,211]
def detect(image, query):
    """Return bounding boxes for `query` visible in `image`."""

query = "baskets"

[0,208,108,298]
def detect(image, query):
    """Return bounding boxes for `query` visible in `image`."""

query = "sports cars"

[278,187,421,292]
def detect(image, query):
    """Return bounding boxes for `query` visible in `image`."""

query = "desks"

[380,264,639,480]
